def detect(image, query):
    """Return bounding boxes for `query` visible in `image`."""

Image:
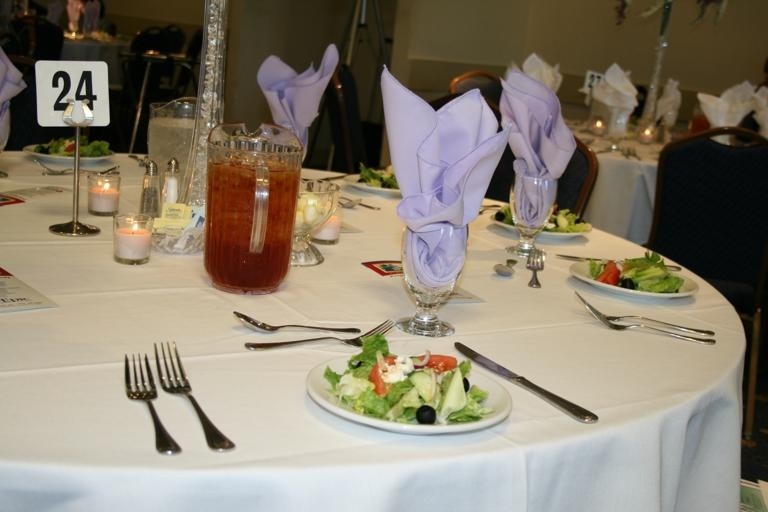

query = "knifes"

[558,252,684,273]
[563,117,664,161]
[453,341,600,426]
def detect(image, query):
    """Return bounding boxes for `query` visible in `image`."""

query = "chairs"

[9,54,49,150]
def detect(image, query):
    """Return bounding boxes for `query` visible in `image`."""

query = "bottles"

[141,158,180,213]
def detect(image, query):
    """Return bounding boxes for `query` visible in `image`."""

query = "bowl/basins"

[153,228,204,254]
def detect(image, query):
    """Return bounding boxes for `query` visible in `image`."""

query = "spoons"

[339,197,362,210]
[235,311,360,334]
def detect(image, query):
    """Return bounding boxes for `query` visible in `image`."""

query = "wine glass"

[290,174,341,269]
[394,170,560,335]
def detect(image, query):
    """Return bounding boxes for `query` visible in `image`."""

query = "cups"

[86,169,154,268]
[148,92,222,196]
[206,121,304,294]
[306,181,340,246]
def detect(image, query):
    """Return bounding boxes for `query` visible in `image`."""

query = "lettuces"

[33,133,113,158]
[357,163,399,188]
[588,253,682,293]
[491,205,590,232]
[324,337,495,425]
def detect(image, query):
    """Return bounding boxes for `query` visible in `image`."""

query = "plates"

[569,260,699,299]
[344,174,400,197]
[23,142,119,166]
[304,352,514,436]
[490,211,595,238]
[63,32,115,44]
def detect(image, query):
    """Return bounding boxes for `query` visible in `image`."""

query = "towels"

[257,45,339,150]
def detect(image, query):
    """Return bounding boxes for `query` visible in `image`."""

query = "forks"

[123,337,234,455]
[573,292,718,347]
[245,321,398,356]
